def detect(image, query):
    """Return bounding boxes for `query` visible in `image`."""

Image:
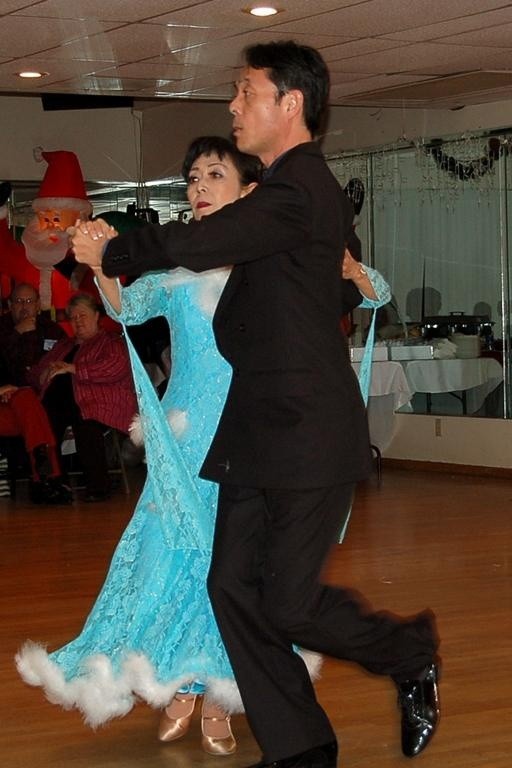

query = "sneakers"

[27,473,119,506]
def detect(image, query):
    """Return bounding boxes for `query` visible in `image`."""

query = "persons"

[17,135,390,754]
[1,284,69,386]
[20,293,139,504]
[1,352,75,505]
[1,146,94,312]
[66,40,440,768]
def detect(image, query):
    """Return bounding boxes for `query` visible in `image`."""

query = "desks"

[400,358,502,416]
[351,361,414,491]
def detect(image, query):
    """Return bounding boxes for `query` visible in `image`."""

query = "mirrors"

[326,135,512,420]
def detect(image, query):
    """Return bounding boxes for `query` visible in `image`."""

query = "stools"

[65,426,130,496]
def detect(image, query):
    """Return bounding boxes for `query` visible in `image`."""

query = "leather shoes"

[394,657,439,757]
[200,694,239,756]
[158,689,197,741]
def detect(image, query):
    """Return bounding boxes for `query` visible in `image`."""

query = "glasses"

[17,298,32,304]
